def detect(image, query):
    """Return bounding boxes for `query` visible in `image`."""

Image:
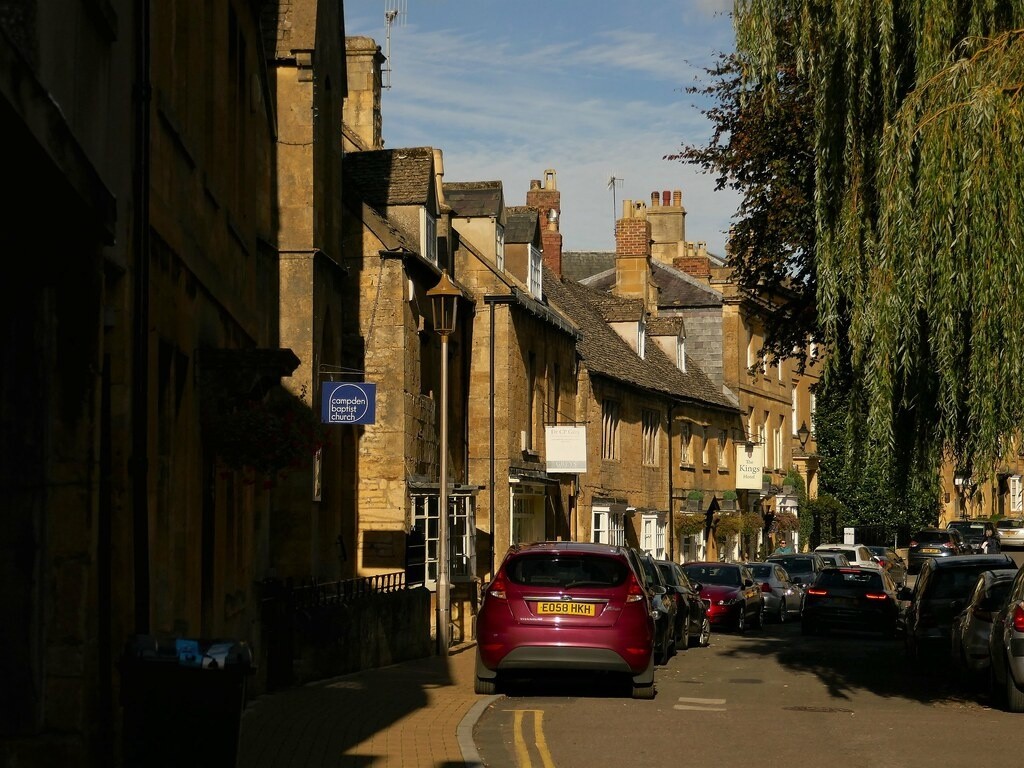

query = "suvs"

[947,520,1002,554]
[765,554,826,591]
[949,569,1018,703]
[474,541,657,700]
[990,564,1023,710]
[910,528,971,571]
[804,565,904,643]
[814,542,882,570]
[637,553,677,664]
[996,518,1024,546]
[895,555,1018,670]
[655,559,710,649]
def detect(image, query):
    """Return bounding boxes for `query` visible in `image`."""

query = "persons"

[775,539,792,554]
[984,529,1001,554]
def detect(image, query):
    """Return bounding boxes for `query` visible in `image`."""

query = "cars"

[715,562,806,622]
[867,546,908,588]
[813,552,850,567]
[680,562,766,630]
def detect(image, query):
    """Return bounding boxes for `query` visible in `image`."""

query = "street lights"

[427,268,461,659]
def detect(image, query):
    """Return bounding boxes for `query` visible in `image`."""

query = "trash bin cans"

[117,636,250,768]
